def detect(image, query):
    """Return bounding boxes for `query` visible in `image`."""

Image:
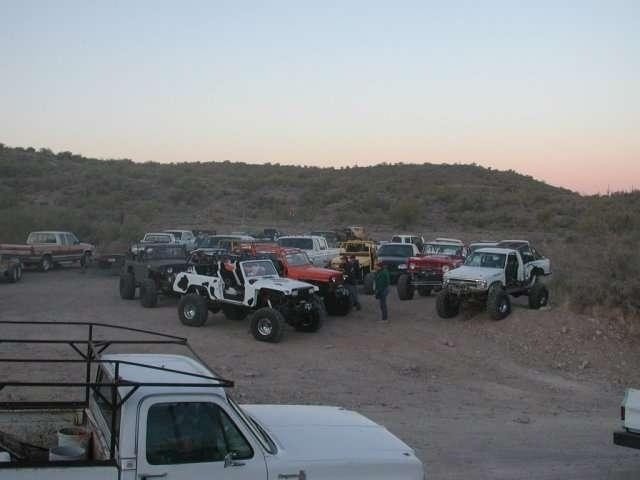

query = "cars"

[611,384,640,452]
[0,257,28,281]
[93,240,137,277]
[428,238,467,257]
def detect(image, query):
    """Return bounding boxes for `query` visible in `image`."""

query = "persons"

[372,262,390,322]
[338,256,350,292]
[348,256,361,310]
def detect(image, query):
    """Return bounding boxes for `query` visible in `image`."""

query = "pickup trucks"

[1,316,425,480]
[0,230,98,273]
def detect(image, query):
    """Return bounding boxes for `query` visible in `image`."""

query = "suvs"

[170,246,327,343]
[397,236,467,301]
[435,238,554,322]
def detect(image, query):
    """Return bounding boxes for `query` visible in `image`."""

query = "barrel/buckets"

[57,426,92,443]
[48,443,87,461]
